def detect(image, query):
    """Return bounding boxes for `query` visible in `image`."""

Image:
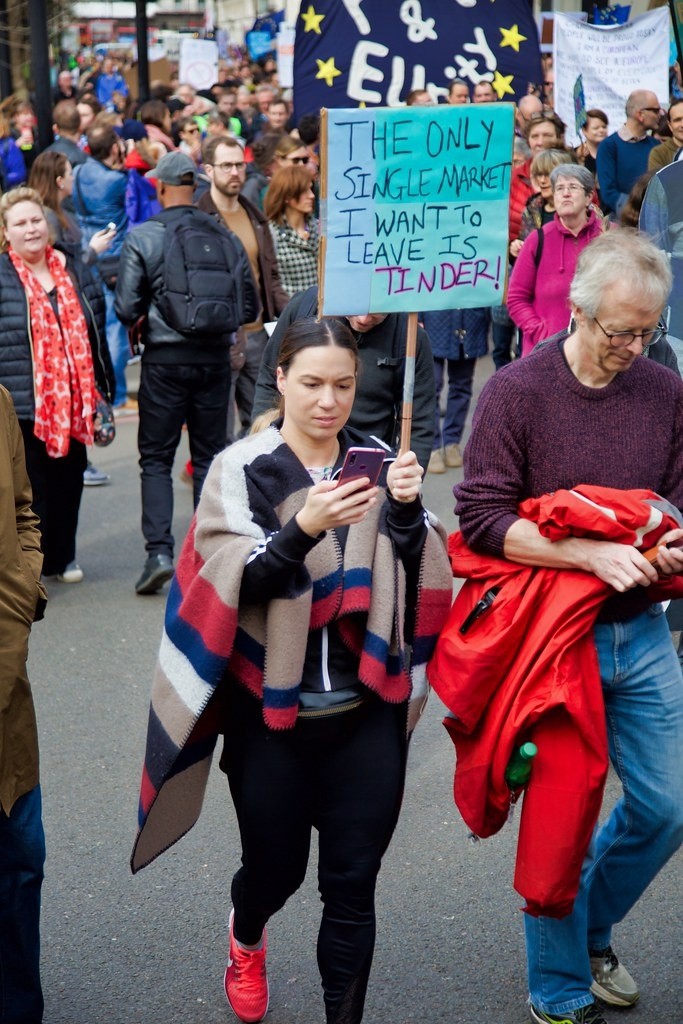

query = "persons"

[0,58,683,417]
[0,386,48,1024]
[179,136,290,495]
[506,164,617,357]
[452,228,683,1024]
[250,286,437,482]
[26,151,109,485]
[0,187,117,583]
[111,151,263,595]
[421,308,489,474]
[131,317,452,1024]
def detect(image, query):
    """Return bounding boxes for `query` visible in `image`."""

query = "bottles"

[505,741,537,790]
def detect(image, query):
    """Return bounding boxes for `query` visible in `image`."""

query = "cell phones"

[334,447,385,506]
[103,222,116,236]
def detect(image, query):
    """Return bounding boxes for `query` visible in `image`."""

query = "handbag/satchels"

[90,388,116,446]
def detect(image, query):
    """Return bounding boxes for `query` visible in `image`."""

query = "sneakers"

[528,1002,608,1024]
[588,946,639,1005]
[223,907,270,1023]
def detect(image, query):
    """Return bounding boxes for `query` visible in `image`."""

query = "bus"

[63,6,210,67]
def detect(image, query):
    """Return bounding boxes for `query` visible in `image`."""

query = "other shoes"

[426,449,446,475]
[443,441,462,468]
[134,553,174,593]
[81,460,110,486]
[57,560,84,582]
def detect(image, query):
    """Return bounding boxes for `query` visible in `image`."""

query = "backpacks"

[146,207,245,336]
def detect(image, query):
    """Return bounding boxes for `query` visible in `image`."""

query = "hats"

[145,151,196,185]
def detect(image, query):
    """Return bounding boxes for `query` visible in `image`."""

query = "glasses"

[591,314,670,349]
[638,108,660,114]
[212,161,245,171]
[283,156,308,165]
[530,111,560,122]
[184,128,201,135]
